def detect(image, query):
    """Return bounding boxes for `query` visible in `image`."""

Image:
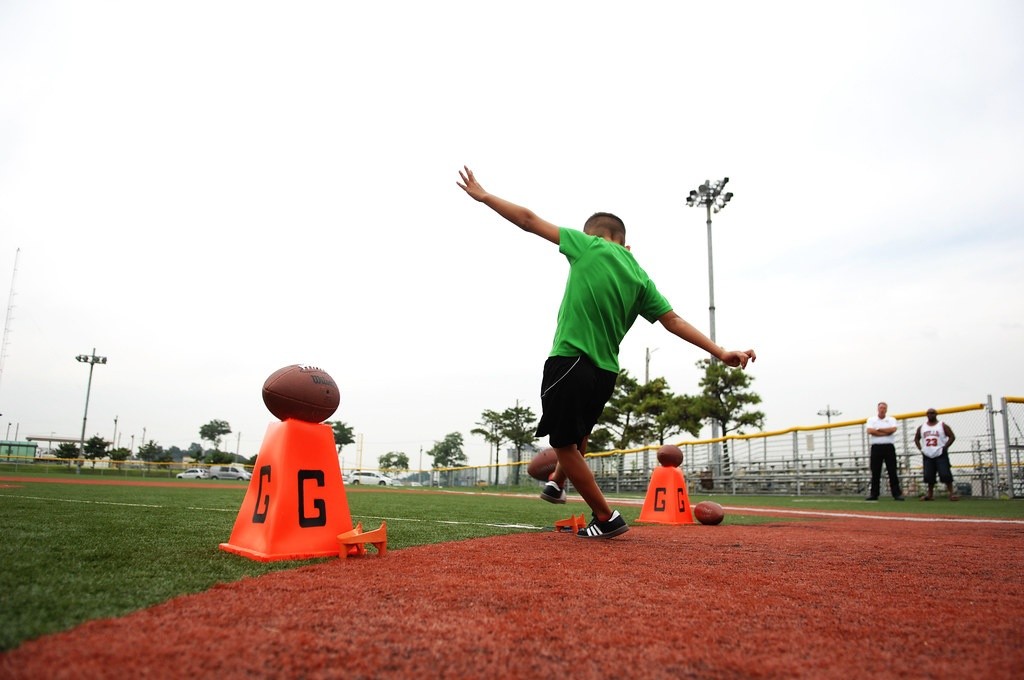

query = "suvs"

[209,465,253,482]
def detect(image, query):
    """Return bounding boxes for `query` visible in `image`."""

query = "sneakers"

[577,509,629,540]
[540,480,566,504]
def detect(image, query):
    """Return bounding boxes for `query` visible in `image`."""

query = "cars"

[343,468,491,489]
[177,466,210,480]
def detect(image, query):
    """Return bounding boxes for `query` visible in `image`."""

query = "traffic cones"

[634,466,694,527]
[213,415,387,567]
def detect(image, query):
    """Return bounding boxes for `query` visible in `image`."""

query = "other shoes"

[919,492,935,500]
[894,495,905,501]
[866,495,878,500]
[949,493,960,500]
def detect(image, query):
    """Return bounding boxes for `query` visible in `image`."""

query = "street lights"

[686,175,728,495]
[75,347,107,466]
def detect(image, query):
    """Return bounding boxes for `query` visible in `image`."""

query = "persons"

[456,164,757,541]
[865,402,906,501]
[914,408,960,501]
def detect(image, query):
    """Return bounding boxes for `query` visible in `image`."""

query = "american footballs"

[693,499,727,525]
[526,447,561,483]
[657,444,684,469]
[261,362,341,425]
